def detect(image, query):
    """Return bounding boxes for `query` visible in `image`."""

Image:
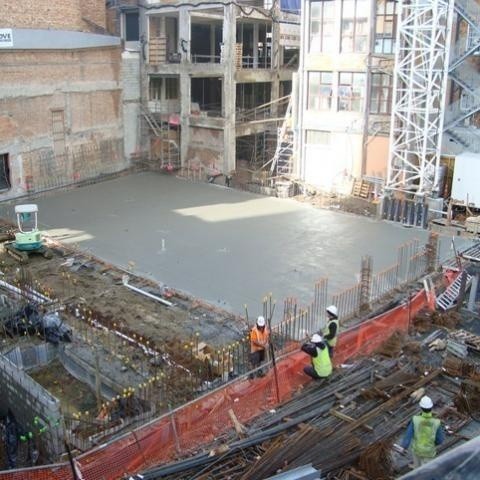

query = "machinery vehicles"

[0,202,56,266]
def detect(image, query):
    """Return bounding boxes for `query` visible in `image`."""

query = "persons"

[399,396,444,468]
[301,334,333,379]
[321,305,339,358]
[248,316,269,382]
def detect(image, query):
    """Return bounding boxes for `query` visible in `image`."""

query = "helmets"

[311,334,323,343]
[257,315,266,327]
[326,305,338,318]
[419,395,433,410]
